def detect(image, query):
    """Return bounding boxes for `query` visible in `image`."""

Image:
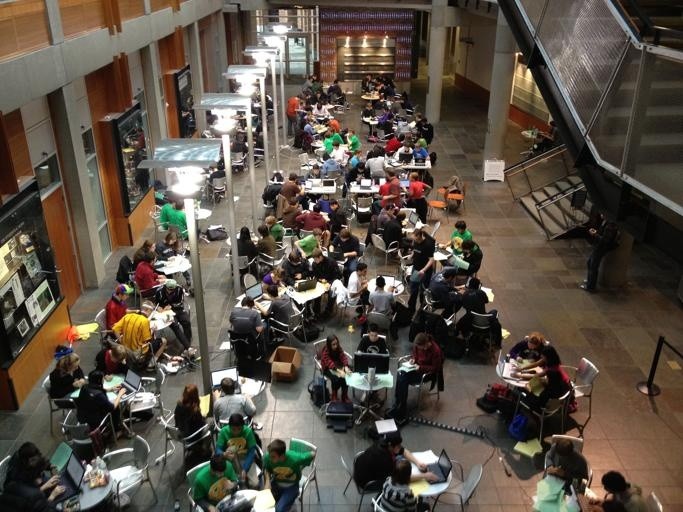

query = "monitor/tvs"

[354,353,390,387]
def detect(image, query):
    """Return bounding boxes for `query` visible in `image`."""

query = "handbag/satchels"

[357,212,372,223]
[310,385,330,406]
[206,228,227,240]
[295,321,319,342]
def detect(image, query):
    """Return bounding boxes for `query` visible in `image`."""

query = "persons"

[192,454,239,512]
[229,72,502,363]
[511,346,577,423]
[356,323,386,394]
[2,441,72,512]
[385,333,442,417]
[262,439,316,512]
[216,414,262,490]
[509,332,552,359]
[321,335,353,404]
[382,458,417,512]
[353,430,439,492]
[76,370,127,441]
[545,439,589,495]
[174,384,212,457]
[201,90,273,204]
[588,469,650,512]
[107,197,198,362]
[212,377,262,449]
[50,344,83,408]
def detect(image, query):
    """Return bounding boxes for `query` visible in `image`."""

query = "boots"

[342,388,352,405]
[331,391,340,402]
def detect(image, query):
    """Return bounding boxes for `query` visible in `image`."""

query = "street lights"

[136,135,225,392]
[199,20,290,303]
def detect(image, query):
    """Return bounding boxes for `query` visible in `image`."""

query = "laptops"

[379,177,387,186]
[399,180,409,192]
[113,368,141,399]
[376,275,395,292]
[323,179,335,193]
[422,448,453,484]
[360,178,373,191]
[293,279,317,292]
[396,154,412,163]
[304,180,313,191]
[403,210,420,229]
[244,282,271,310]
[308,179,321,187]
[329,252,347,261]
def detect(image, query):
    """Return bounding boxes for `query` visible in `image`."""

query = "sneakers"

[147,363,160,371]
[581,285,593,292]
[386,405,400,416]
[395,408,407,423]
[187,347,197,356]
[123,431,135,438]
[583,279,591,284]
[181,350,189,359]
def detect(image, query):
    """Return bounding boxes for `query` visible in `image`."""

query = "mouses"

[289,288,293,291]
[395,289,398,293]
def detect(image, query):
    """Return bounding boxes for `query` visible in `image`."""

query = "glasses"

[332,343,338,345]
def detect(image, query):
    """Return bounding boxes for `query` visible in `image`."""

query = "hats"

[555,438,575,461]
[166,279,176,289]
[115,284,134,294]
